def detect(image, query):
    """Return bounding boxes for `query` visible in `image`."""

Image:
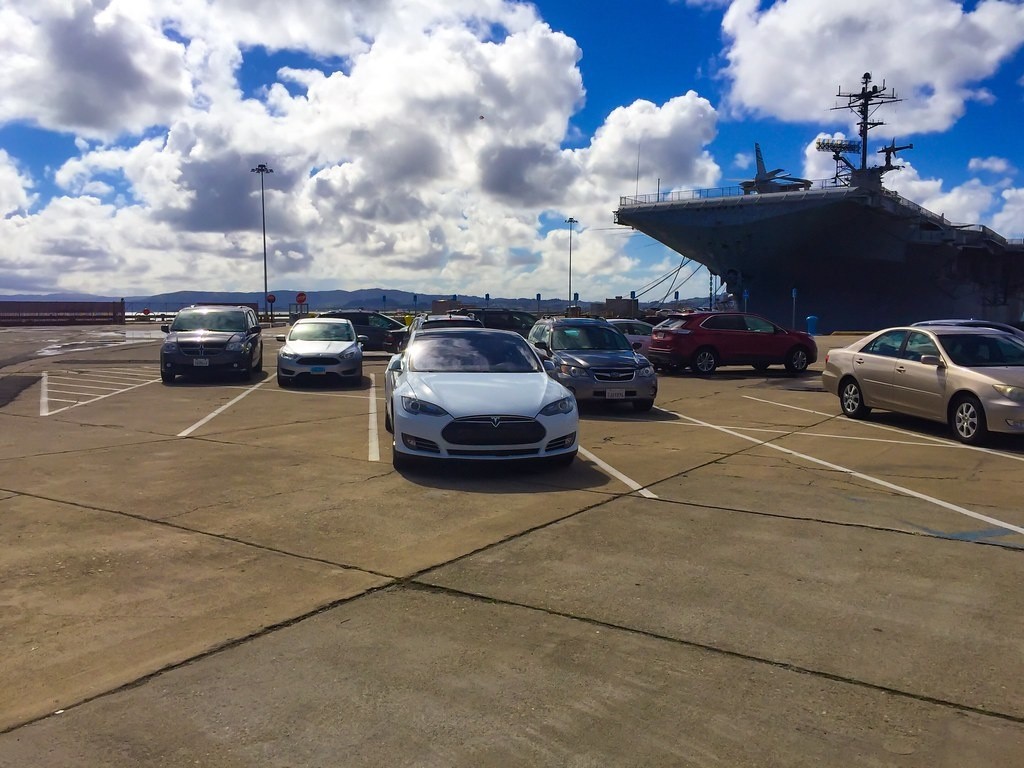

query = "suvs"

[316,309,406,351]
[409,313,485,338]
[527,314,658,411]
[160,306,263,382]
[648,308,818,376]
[448,308,540,340]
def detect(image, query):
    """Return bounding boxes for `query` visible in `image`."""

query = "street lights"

[565,218,578,311]
[251,164,275,316]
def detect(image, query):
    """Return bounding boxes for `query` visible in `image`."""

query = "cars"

[604,318,655,359]
[275,319,369,386]
[822,326,1024,444]
[385,325,411,354]
[383,328,579,468]
[911,319,1024,344]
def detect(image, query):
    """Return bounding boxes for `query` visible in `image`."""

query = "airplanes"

[727,143,813,193]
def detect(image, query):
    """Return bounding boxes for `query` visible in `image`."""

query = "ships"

[617,68,1024,335]
